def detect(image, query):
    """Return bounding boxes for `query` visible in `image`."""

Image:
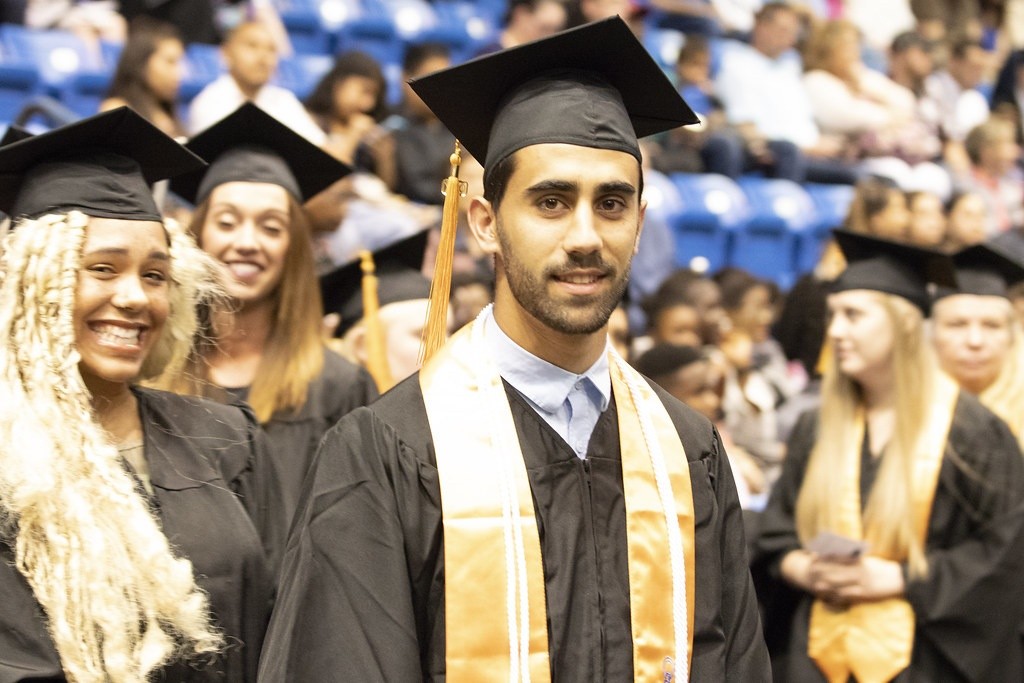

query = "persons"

[1,0,1024,332]
[929,234,1023,451]
[165,105,382,544]
[252,12,776,683]
[611,269,789,511]
[744,230,1024,683]
[2,102,292,682]
[321,225,449,393]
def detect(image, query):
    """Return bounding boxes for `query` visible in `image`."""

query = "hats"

[307,221,435,392]
[0,105,212,230]
[406,14,702,384]
[823,227,949,320]
[930,244,1024,306]
[168,101,356,207]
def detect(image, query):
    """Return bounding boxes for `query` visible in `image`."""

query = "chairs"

[0,0,858,334]
